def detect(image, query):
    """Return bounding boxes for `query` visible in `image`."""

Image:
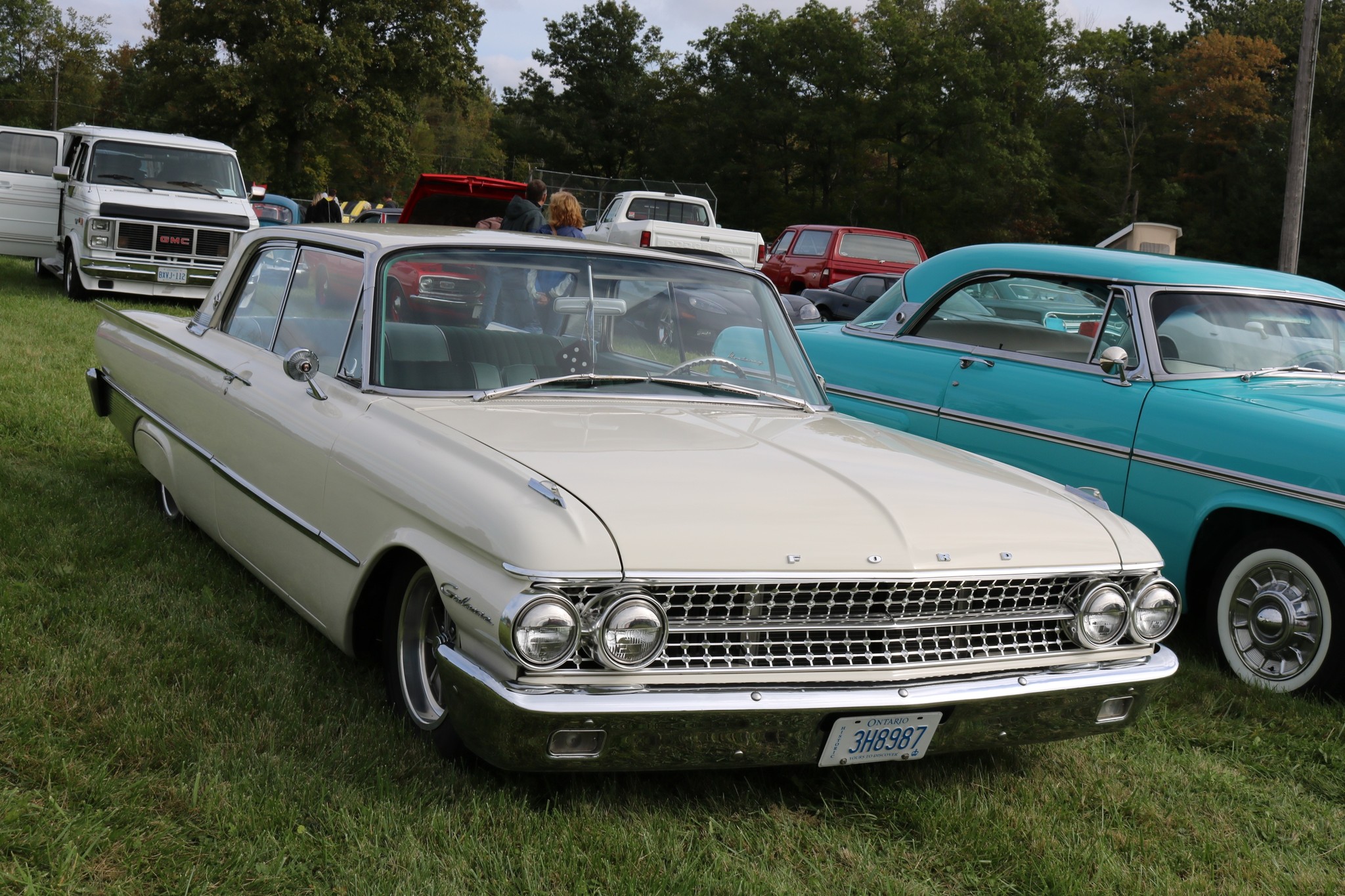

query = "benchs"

[245,316,570,390]
[922,317,1112,359]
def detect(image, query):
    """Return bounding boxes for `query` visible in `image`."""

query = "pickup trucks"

[582,191,766,271]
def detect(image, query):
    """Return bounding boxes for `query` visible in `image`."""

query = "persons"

[363,202,371,211]
[444,208,502,328]
[304,189,342,223]
[497,179,587,337]
[381,190,399,208]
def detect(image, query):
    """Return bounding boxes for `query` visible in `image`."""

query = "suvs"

[0,122,261,307]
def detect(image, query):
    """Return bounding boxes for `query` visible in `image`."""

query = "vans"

[759,225,929,297]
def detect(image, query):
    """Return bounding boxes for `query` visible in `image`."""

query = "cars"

[244,193,383,267]
[710,245,1345,699]
[603,246,1128,347]
[84,223,1170,777]
[306,173,526,324]
[1156,300,1345,372]
